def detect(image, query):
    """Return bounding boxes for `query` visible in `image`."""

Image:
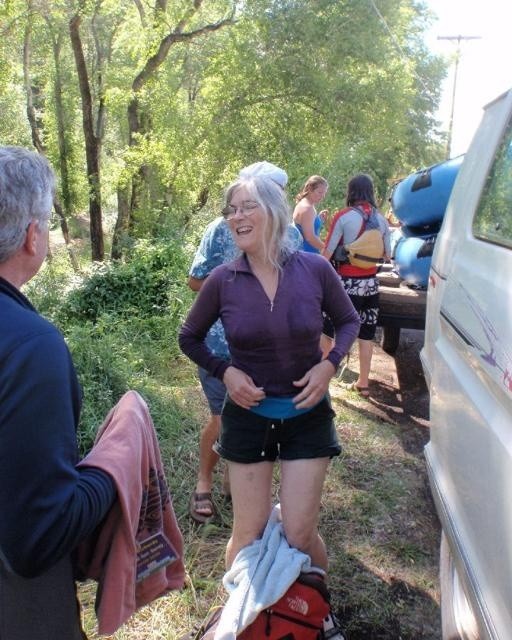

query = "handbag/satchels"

[247,396,313,419]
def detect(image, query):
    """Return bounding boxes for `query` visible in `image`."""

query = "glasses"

[36,212,66,231]
[221,200,261,221]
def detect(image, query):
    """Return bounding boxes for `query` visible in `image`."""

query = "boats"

[388,151,467,291]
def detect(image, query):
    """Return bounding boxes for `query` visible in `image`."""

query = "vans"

[419,86,512,640]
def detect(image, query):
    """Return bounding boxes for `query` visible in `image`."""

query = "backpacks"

[175,572,332,640]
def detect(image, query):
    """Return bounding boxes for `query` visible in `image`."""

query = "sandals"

[337,383,370,399]
[189,490,215,521]
[322,608,347,640]
[219,491,232,501]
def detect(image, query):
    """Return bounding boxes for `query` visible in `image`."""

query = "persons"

[1,145,150,639]
[178,172,362,571]
[186,160,306,527]
[291,174,401,399]
